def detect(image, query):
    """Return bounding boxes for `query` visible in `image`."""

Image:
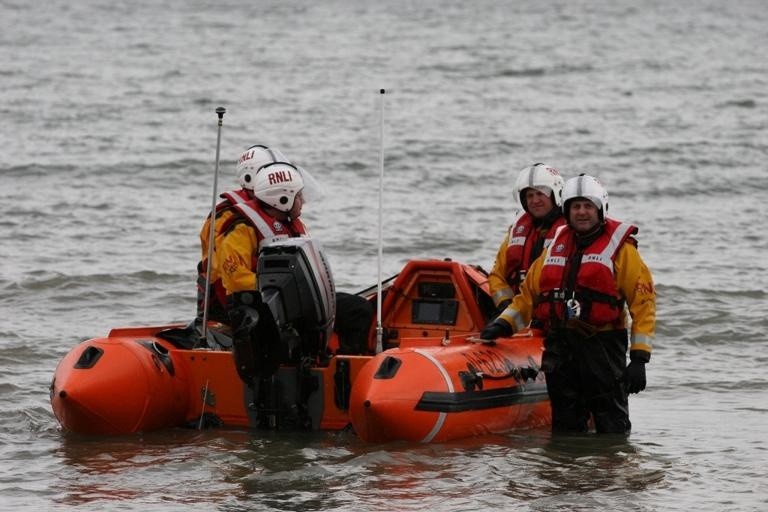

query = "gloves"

[480,324,510,347]
[617,358,646,393]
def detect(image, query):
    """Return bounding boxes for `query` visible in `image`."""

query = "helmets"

[561,174,610,227]
[235,144,292,191]
[512,162,565,212]
[253,162,326,213]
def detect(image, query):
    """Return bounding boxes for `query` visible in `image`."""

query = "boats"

[44,256,556,445]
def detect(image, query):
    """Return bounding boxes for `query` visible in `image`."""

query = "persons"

[480,168,659,441]
[194,143,291,329]
[487,160,566,312]
[218,160,336,447]
